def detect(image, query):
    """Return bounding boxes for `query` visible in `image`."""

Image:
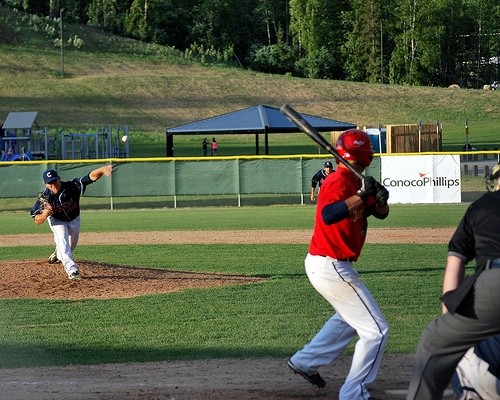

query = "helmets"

[335,129,374,167]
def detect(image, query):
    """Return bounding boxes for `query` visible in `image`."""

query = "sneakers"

[287,360,326,389]
[68,271,83,280]
[48,252,59,264]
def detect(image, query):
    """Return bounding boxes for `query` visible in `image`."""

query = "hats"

[490,162,500,180]
[43,169,59,183]
[324,161,333,168]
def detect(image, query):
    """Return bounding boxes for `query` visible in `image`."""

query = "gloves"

[374,182,389,207]
[356,176,376,203]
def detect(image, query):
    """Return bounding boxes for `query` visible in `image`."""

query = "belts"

[320,255,354,262]
[476,258,500,273]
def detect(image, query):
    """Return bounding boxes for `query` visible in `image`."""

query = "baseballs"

[121,134,129,143]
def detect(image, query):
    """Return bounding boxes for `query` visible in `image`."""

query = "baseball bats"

[277,102,387,204]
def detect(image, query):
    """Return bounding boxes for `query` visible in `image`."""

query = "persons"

[203,138,218,156]
[285,128,389,400]
[30,164,112,280]
[407,161,500,400]
[311,161,336,201]
